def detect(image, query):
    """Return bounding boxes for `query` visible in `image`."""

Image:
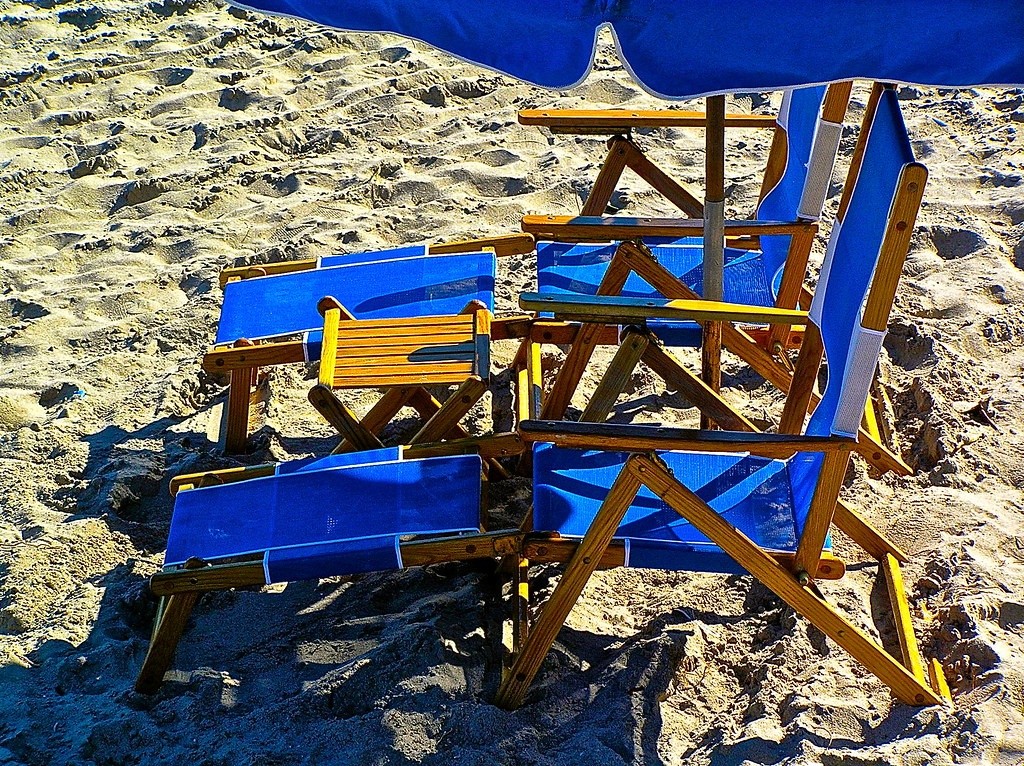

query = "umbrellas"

[226,0,1024,429]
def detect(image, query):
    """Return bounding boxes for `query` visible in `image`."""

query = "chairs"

[200,79,920,475]
[119,83,957,708]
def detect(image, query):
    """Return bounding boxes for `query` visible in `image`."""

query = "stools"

[308,295,512,484]
